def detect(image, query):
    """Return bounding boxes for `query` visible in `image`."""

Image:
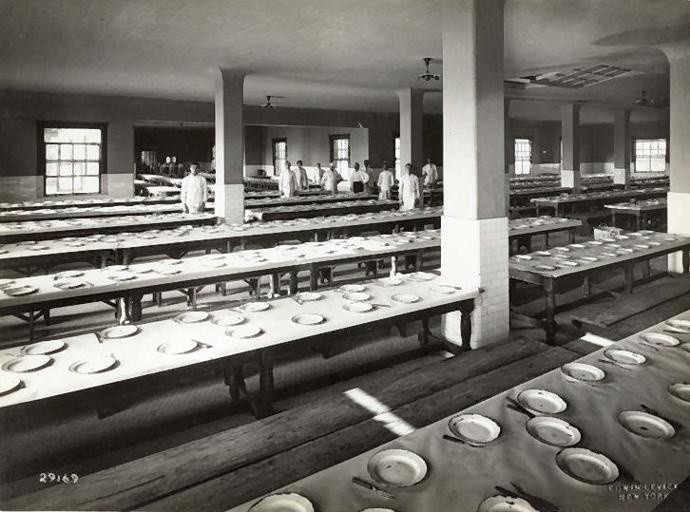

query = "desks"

[0,173,690,512]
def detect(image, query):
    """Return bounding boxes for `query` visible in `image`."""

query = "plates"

[0,272,453,396]
[1,230,98,254]
[100,224,439,271]
[514,231,678,274]
[507,216,570,232]
[259,198,440,224]
[247,317,689,512]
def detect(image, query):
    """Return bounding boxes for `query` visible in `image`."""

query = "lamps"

[417,58,440,84]
[259,95,277,112]
[632,91,660,108]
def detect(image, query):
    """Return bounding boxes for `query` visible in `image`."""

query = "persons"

[397,163,420,211]
[348,162,370,193]
[293,160,309,191]
[311,162,326,185]
[318,158,342,193]
[277,160,298,199]
[180,162,209,213]
[361,160,375,191]
[376,163,396,199]
[421,157,439,186]
